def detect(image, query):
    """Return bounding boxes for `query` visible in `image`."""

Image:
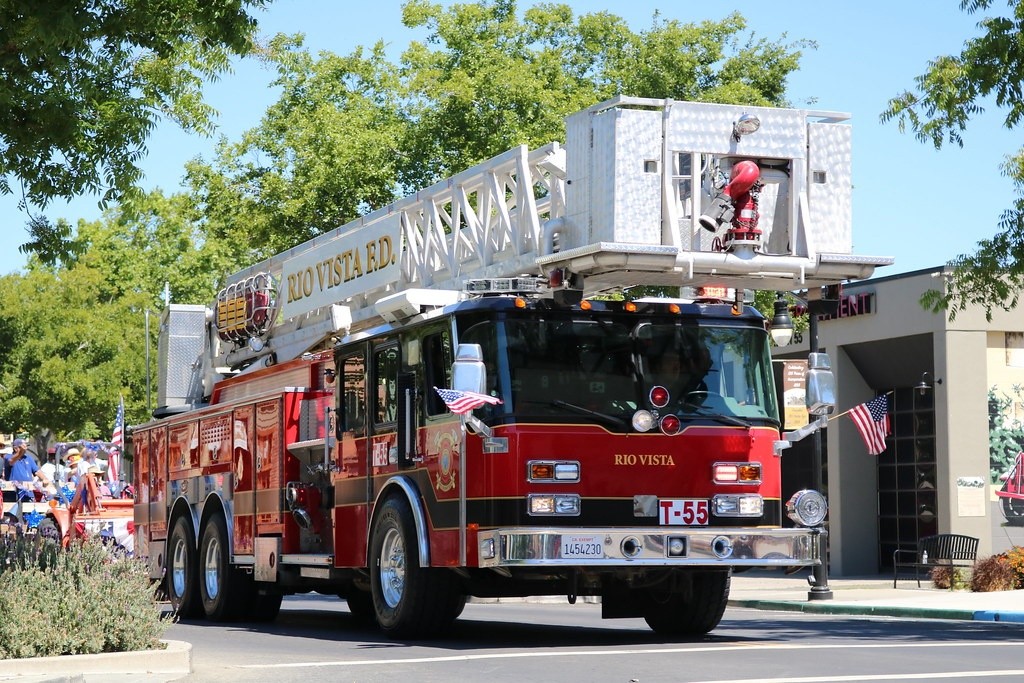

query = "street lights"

[771,282,839,600]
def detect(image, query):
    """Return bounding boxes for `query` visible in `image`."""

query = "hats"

[63,448,82,462]
[13,438,26,447]
[48,447,55,454]
[0,434,4,444]
[89,465,106,477]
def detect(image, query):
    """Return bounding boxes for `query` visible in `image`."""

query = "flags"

[105,404,122,482]
[848,395,892,455]
[435,389,504,415]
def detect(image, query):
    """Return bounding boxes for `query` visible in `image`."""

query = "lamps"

[915,372,942,395]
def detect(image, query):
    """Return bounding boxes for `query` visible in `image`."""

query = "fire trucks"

[127,91,895,643]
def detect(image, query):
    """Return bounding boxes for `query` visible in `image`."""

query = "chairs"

[893,533,979,591]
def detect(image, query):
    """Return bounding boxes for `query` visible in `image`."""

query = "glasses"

[0,444,6,447]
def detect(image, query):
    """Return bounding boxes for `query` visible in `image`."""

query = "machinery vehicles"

[37,440,136,554]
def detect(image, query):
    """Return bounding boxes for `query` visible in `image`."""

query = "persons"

[0,438,108,503]
